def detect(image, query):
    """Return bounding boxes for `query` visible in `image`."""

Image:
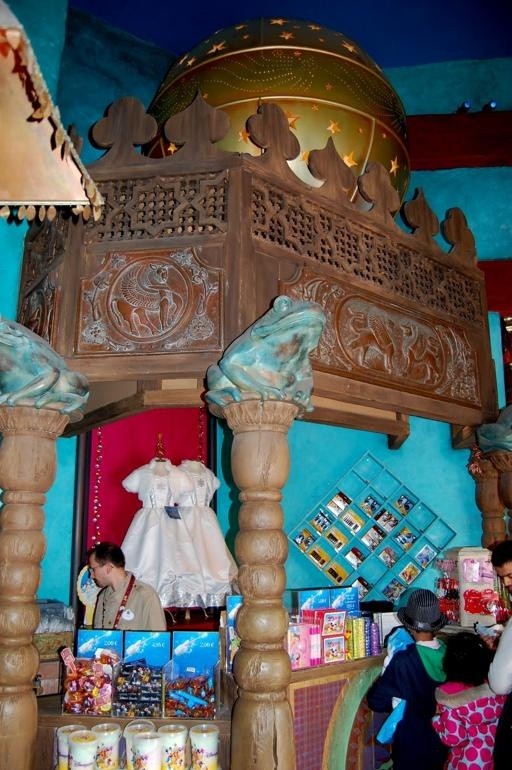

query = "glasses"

[89,563,108,574]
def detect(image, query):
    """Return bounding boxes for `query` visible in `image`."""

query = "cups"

[56,718,220,770]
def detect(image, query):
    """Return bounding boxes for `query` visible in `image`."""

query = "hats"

[396,588,448,633]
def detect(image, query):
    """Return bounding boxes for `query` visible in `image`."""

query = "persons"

[88,541,167,632]
[119,459,240,609]
[366,538,512,770]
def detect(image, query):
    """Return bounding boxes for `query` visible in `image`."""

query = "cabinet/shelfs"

[287,449,458,602]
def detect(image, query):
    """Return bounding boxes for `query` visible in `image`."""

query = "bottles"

[346,615,381,659]
